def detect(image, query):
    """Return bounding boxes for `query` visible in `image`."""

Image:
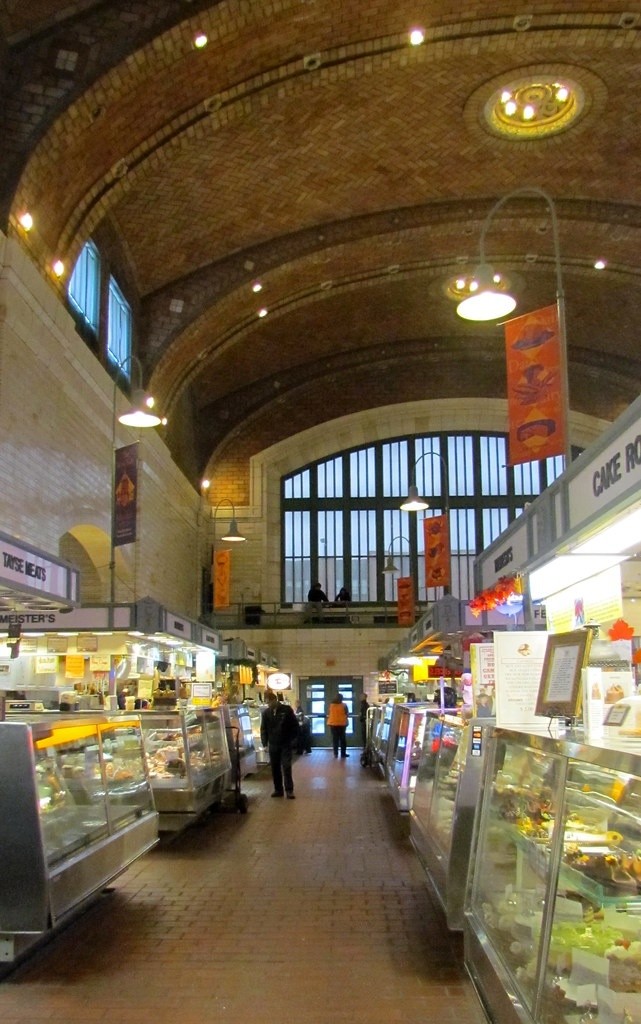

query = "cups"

[106,695,117,710]
[124,696,136,710]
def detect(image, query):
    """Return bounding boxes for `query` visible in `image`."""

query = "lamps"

[212,498,245,551]
[104,356,163,627]
[455,187,573,469]
[400,451,452,596]
[381,536,417,625]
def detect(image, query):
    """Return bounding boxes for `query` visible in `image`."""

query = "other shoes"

[334,755,338,758]
[341,753,349,757]
[304,750,312,754]
[295,752,303,755]
[271,792,284,797]
[286,791,295,799]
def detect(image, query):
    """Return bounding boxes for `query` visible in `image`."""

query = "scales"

[5,700,43,712]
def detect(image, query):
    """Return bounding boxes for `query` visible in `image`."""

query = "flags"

[504,303,565,466]
[114,442,139,547]
[213,550,231,607]
[397,577,415,625]
[423,516,450,586]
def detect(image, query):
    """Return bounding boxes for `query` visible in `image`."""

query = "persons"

[326,693,350,758]
[334,588,351,601]
[357,692,370,756]
[308,582,330,603]
[261,692,299,799]
[294,699,311,756]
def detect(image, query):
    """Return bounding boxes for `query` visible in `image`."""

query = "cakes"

[485,781,641,1024]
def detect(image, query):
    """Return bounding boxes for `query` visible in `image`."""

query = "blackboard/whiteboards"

[377,680,398,695]
